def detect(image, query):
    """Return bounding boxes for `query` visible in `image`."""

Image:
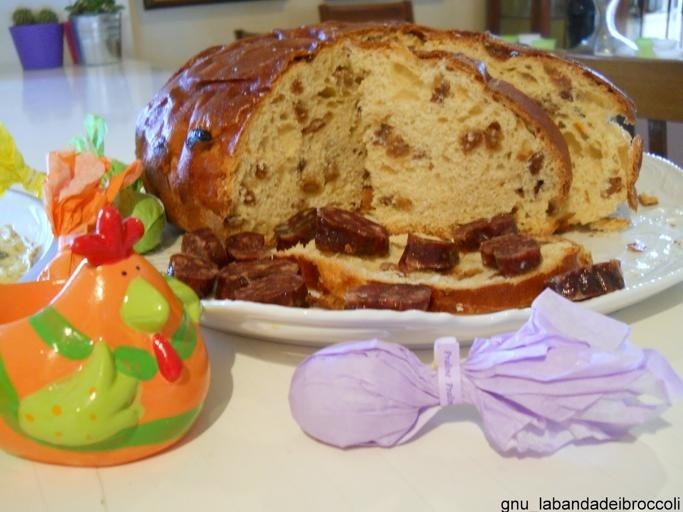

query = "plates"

[101,143,680,360]
[0,183,64,285]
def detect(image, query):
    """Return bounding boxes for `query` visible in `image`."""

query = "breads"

[132,20,645,316]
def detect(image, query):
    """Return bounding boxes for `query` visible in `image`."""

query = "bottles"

[561,1,640,61]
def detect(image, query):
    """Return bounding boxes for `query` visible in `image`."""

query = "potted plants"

[8,0,124,72]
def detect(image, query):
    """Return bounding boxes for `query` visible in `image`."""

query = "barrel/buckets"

[67,12,124,65]
